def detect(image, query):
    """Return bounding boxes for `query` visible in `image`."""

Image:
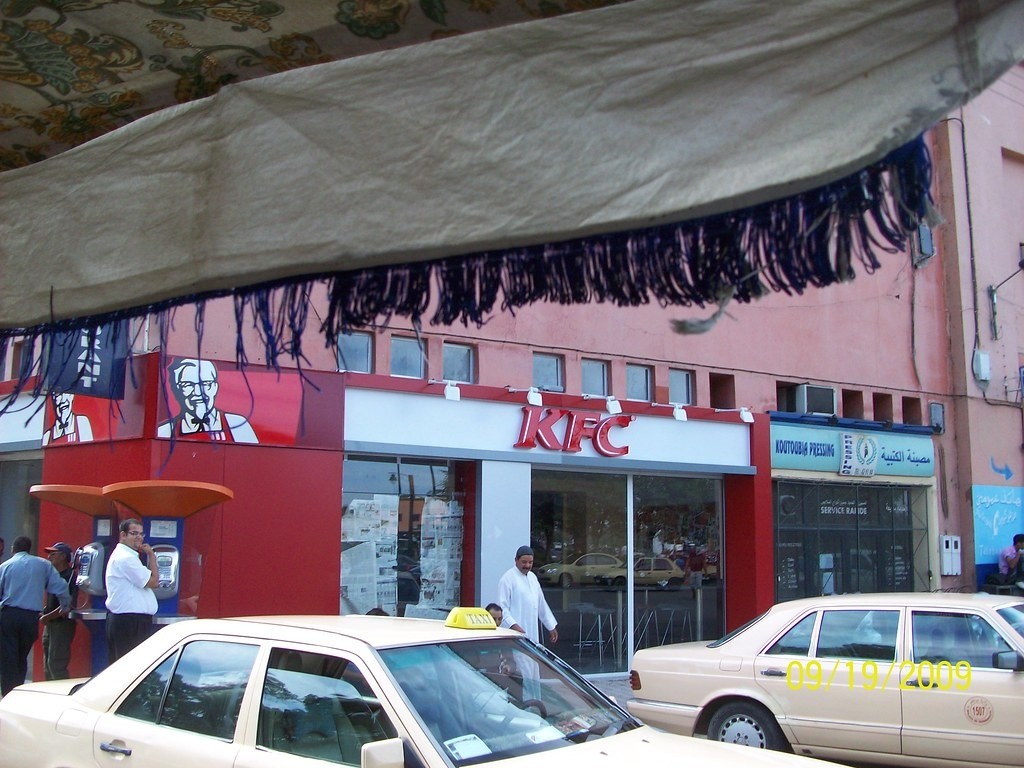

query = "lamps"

[652,402,688,421]
[509,387,542,407]
[584,395,622,414]
[855,420,893,429]
[428,378,460,400]
[905,424,942,433]
[715,407,754,423]
[802,414,838,425]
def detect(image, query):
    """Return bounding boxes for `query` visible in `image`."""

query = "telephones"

[72,541,110,597]
[146,544,180,600]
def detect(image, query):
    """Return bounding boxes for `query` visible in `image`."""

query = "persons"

[105,518,159,664]
[685,545,708,600]
[0,536,71,697]
[40,542,78,681]
[471,603,523,700]
[342,609,414,703]
[498,545,558,717]
[999,534,1024,589]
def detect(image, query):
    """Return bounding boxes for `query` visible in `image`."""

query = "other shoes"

[1015,582,1024,589]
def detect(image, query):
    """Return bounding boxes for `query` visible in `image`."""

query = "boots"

[692,589,695,599]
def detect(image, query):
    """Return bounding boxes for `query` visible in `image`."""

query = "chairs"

[817,622,993,666]
[180,664,462,768]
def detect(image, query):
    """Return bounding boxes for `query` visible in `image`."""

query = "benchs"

[982,585,1017,596]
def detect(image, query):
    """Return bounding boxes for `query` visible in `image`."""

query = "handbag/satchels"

[985,574,1005,585]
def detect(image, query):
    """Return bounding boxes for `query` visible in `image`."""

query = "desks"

[590,586,709,669]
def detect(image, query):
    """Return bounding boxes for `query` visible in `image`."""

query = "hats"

[516,546,534,557]
[43,542,72,551]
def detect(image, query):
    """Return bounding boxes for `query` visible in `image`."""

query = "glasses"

[126,532,145,537]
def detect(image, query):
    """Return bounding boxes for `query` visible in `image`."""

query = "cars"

[594,557,686,593]
[537,552,624,589]
[624,592,1024,768]
[1,607,853,768]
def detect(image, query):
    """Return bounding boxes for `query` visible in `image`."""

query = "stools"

[579,605,693,666]
